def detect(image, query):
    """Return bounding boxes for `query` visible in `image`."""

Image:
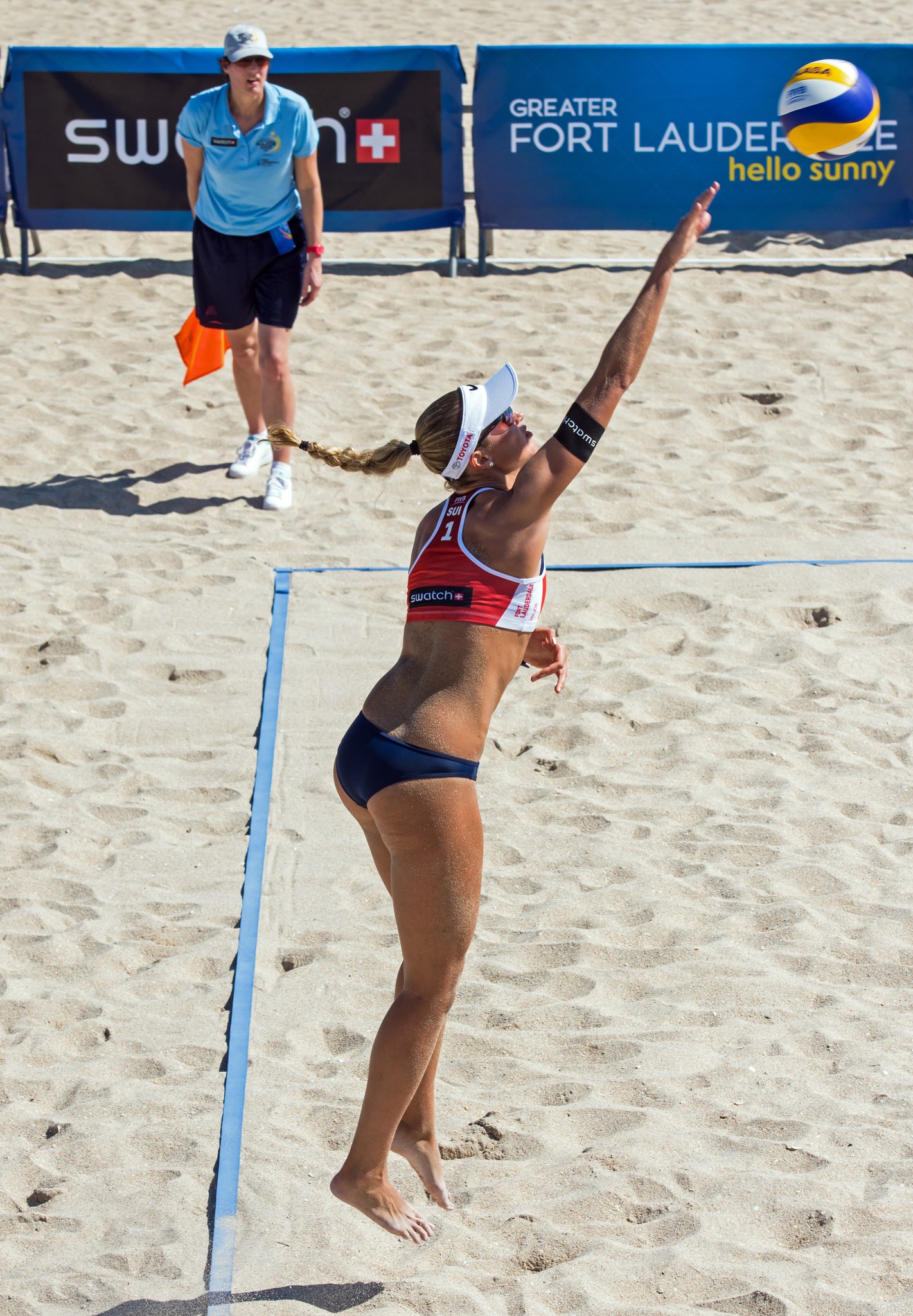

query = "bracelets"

[553,403,605,464]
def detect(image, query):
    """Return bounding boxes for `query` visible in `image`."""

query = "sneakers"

[263,467,292,511]
[228,435,273,478]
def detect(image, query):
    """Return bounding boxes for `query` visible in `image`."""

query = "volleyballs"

[777,58,881,161]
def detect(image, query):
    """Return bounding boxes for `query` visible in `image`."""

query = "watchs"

[305,244,324,256]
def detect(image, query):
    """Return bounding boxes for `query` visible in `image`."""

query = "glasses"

[477,405,514,447]
[235,56,270,68]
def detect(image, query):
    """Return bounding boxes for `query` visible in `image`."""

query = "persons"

[176,26,325,512]
[258,182,724,1246]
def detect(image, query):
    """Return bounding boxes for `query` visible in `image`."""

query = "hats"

[223,24,274,62]
[441,362,518,482]
